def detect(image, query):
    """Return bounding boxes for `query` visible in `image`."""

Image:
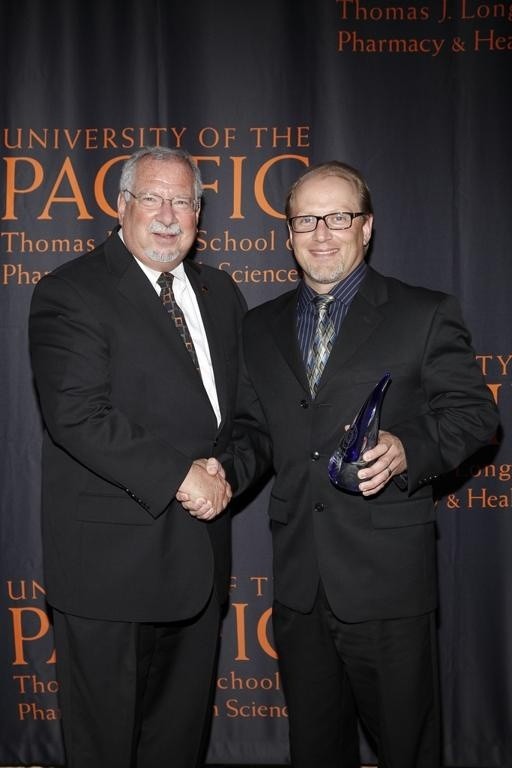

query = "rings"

[385,465,394,475]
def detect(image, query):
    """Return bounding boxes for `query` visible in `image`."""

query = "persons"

[171,161,501,767]
[28,146,253,767]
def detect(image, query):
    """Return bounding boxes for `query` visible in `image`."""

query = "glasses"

[288,212,363,233]
[124,189,199,212]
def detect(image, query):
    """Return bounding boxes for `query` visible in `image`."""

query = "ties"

[158,272,202,380]
[306,293,337,398]
